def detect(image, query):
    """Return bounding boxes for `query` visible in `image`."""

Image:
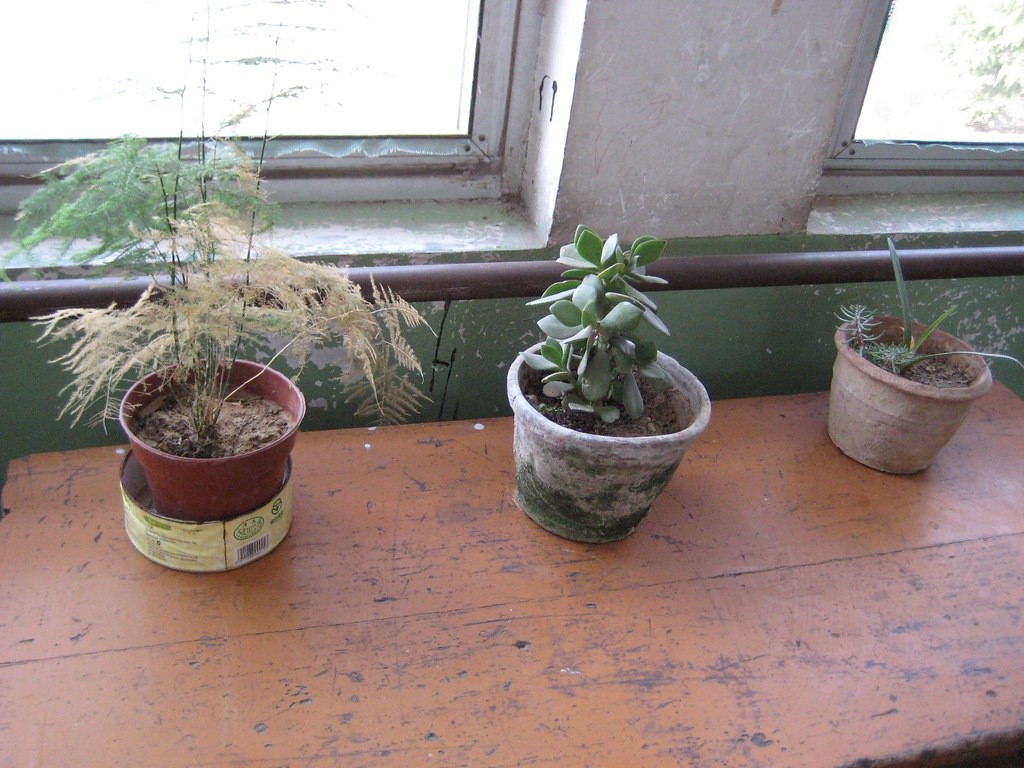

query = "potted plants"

[0,0,439,522]
[827,238,1024,475]
[507,221,712,544]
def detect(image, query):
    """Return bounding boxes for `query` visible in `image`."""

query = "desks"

[0,376,1024,768]
